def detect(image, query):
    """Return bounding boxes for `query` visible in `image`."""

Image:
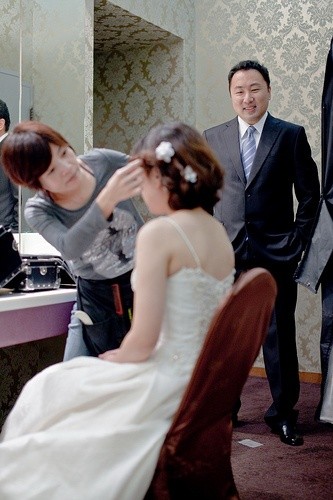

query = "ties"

[241,126,255,182]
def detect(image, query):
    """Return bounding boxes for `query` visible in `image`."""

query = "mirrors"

[0,70,34,232]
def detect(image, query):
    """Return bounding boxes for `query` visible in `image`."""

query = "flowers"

[179,165,197,183]
[154,140,174,163]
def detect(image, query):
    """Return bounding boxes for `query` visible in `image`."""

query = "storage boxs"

[12,258,61,292]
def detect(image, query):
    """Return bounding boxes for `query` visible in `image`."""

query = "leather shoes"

[270,422,303,445]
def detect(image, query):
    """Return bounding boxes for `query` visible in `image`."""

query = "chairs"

[141,267,277,500]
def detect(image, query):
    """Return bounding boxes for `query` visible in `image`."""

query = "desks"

[0,288,79,349]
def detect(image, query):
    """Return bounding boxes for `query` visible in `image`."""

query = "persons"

[201,60,321,445]
[0,123,235,500]
[0,100,22,233]
[0,121,148,358]
